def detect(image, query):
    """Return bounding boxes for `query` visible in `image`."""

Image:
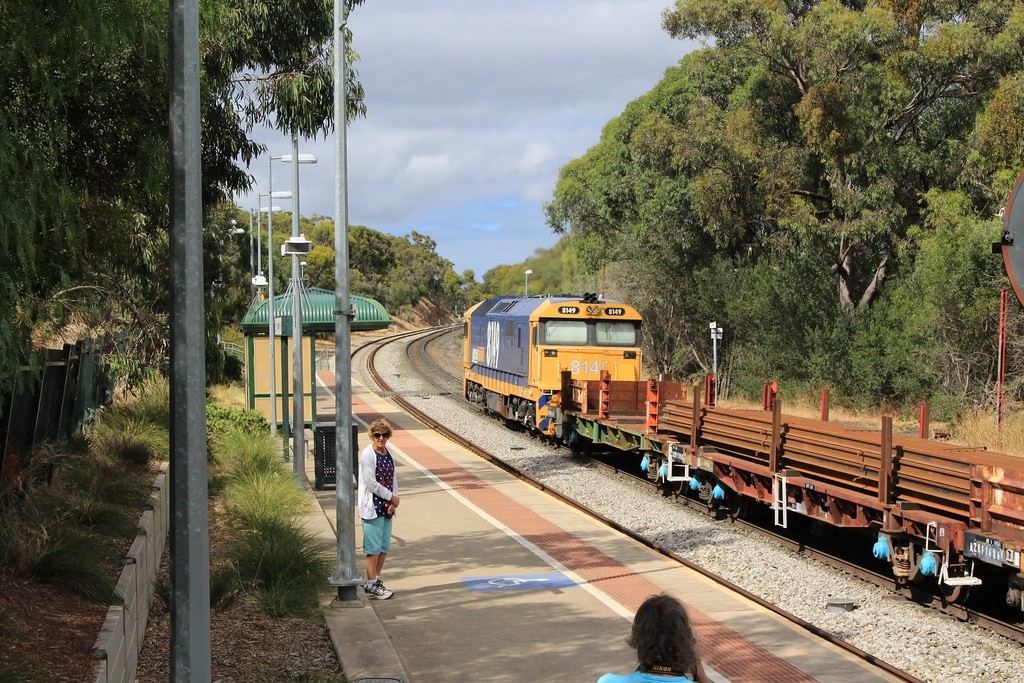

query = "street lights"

[525,269,533,297]
[269,155,317,442]
[256,191,292,303]
[249,207,282,304]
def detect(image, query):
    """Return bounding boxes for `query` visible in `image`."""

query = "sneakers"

[364,579,394,598]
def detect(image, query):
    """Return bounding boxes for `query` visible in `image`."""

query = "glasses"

[374,432,389,436]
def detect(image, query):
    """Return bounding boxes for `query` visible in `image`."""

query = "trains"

[464,298,1024,620]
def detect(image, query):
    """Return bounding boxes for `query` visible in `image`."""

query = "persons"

[595,591,715,683]
[358,419,399,600]
